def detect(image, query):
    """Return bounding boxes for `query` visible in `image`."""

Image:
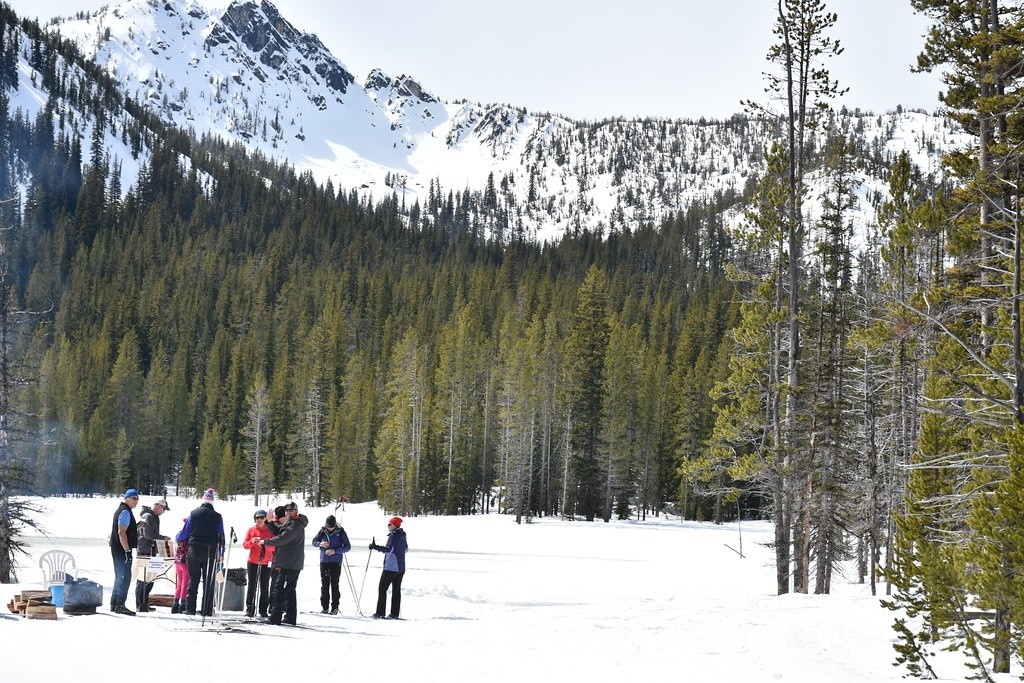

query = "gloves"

[124,550,132,565]
[369,544,380,550]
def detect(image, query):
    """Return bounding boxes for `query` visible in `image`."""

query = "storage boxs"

[47,584,65,607]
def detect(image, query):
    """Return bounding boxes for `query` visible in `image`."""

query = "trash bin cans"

[218,569,246,611]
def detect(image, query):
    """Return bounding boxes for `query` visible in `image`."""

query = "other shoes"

[110,599,298,627]
[387,614,399,619]
[329,609,338,615]
[322,607,329,613]
[370,614,385,619]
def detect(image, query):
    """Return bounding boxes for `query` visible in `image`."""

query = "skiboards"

[182,616,270,624]
[300,610,354,620]
[158,623,257,633]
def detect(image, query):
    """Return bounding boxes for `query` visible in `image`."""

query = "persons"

[110,489,139,615]
[312,515,352,615]
[135,499,171,612]
[243,502,309,625]
[176,488,225,615]
[171,519,189,613]
[369,517,409,619]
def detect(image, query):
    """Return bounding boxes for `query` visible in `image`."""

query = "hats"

[325,515,337,528]
[154,498,170,511]
[284,502,299,512]
[201,488,215,507]
[275,506,285,518]
[389,517,403,527]
[125,490,138,499]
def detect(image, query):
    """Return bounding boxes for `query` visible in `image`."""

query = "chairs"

[40,550,81,591]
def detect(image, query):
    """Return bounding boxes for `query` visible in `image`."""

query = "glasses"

[255,515,265,520]
[388,524,394,527]
[161,505,166,512]
[276,516,282,520]
[284,509,296,513]
[128,496,139,500]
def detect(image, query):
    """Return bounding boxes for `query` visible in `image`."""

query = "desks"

[137,556,177,613]
